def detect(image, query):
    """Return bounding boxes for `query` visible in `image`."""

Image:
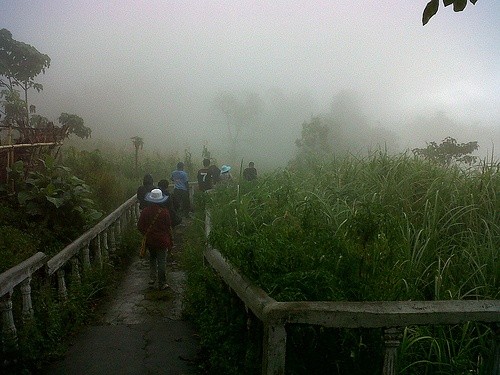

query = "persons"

[138,175,182,290]
[220,165,233,182]
[243,162,257,181]
[198,158,221,193]
[169,162,191,218]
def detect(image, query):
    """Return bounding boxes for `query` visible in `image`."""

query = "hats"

[143,188,168,203]
[220,164,231,174]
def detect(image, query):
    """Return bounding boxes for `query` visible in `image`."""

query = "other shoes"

[148,280,155,284]
[136,235,146,258]
[159,284,169,290]
